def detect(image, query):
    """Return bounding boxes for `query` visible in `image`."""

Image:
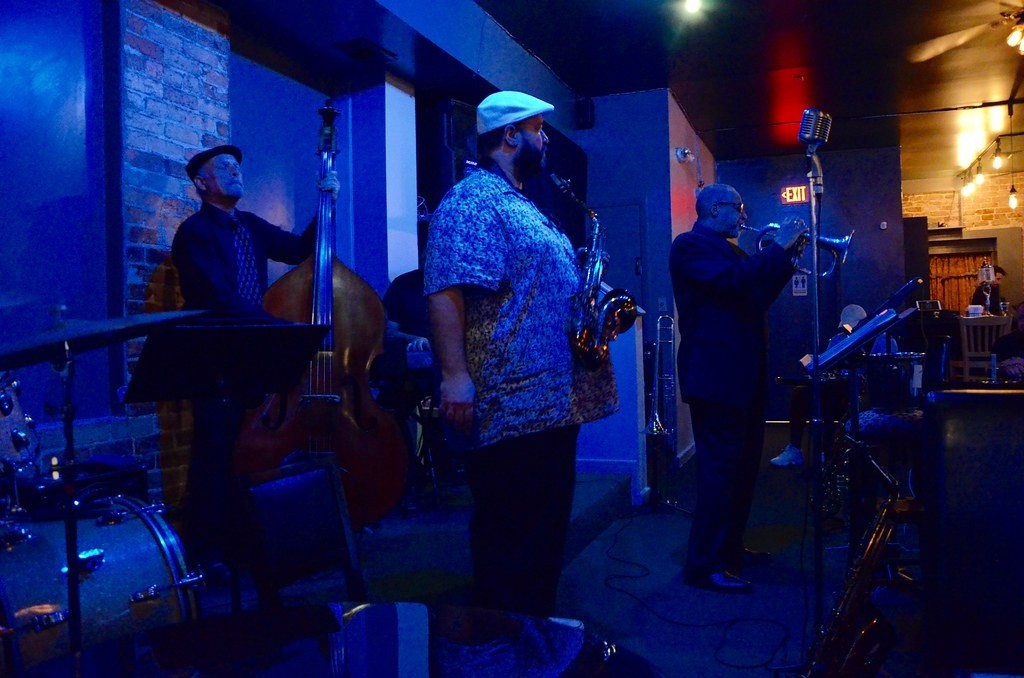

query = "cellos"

[233,97,408,530]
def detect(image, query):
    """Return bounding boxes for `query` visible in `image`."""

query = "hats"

[837,304,867,328]
[477,91,555,134]
[186,145,243,180]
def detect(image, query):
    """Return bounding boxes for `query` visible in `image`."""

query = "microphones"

[798,108,832,146]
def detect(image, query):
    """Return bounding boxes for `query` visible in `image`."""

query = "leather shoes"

[723,546,772,562]
[687,570,751,590]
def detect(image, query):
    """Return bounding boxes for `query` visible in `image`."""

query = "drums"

[0,366,42,476]
[0,494,209,676]
[857,351,925,419]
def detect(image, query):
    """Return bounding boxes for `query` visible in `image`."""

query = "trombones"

[636,315,679,509]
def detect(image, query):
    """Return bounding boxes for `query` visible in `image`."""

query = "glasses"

[719,201,744,213]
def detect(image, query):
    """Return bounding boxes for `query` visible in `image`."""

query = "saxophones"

[547,168,637,366]
[799,435,904,678]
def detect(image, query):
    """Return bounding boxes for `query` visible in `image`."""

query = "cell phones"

[916,300,942,311]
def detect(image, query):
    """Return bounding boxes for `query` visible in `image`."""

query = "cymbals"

[0,309,207,370]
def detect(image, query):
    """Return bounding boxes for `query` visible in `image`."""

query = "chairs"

[949,315,1013,381]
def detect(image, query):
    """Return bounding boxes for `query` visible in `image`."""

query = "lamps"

[992,138,1004,169]
[967,168,975,192]
[1008,117,1018,208]
[962,171,968,197]
[976,158,984,185]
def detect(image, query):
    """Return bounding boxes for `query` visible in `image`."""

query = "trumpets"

[739,224,854,280]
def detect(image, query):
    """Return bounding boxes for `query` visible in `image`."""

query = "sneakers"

[771,443,805,466]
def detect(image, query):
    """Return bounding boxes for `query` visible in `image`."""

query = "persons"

[383,222,478,509]
[986,302,1024,378]
[169,145,340,545]
[422,91,620,618]
[966,265,1005,351]
[669,184,810,594]
[769,304,898,467]
[794,276,806,288]
